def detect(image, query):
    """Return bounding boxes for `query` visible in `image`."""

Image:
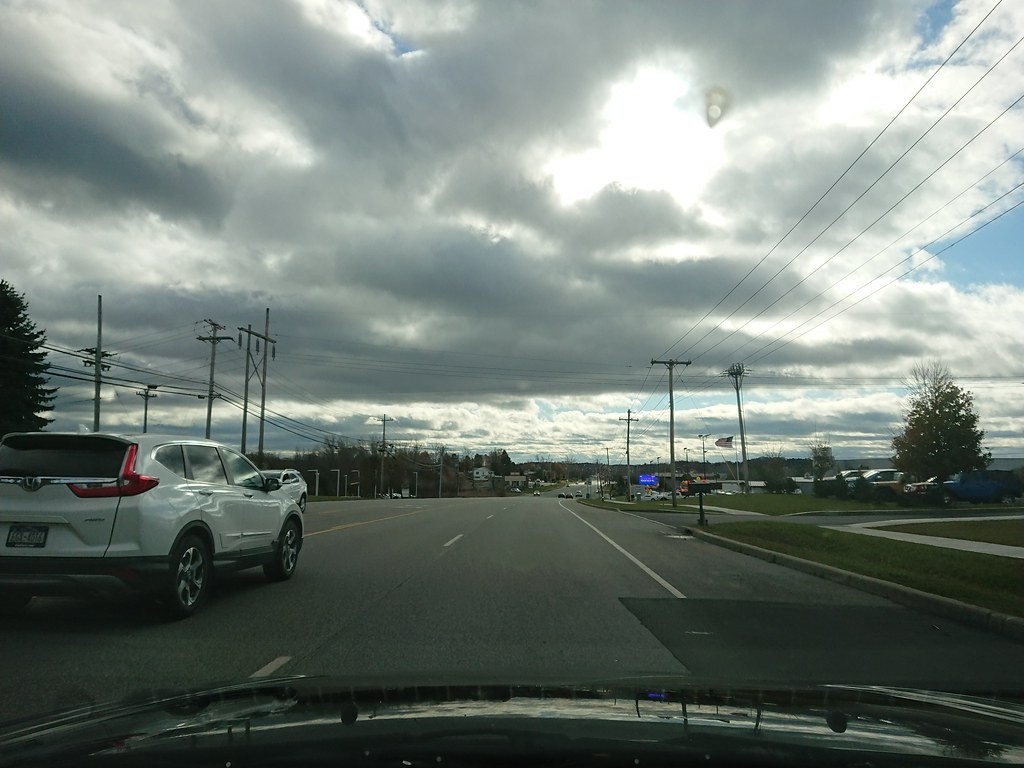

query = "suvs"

[576,491,583,498]
[903,470,1023,506]
[858,469,934,501]
[259,468,307,518]
[0,428,304,628]
[659,480,810,501]
[825,470,868,491]
[534,490,540,496]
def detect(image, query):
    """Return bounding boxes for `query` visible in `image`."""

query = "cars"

[566,493,574,499]
[558,493,566,498]
[530,476,612,493]
[635,493,659,502]
[511,488,521,494]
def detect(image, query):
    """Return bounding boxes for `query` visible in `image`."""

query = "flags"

[715,436,733,448]
